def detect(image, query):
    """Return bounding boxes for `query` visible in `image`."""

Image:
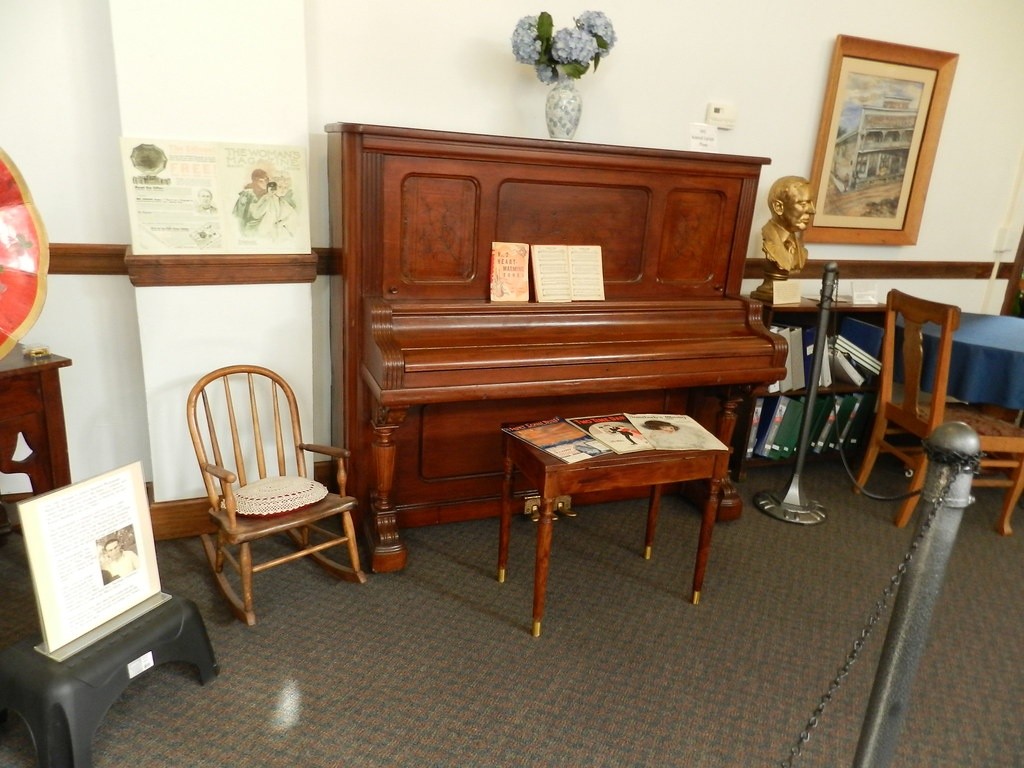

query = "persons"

[610,427,637,446]
[761,175,816,272]
[644,419,701,448]
[99,539,139,581]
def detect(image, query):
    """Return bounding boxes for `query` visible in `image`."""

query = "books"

[500,413,728,464]
[491,241,605,303]
[745,315,884,461]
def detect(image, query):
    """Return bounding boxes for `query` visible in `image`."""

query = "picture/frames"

[796,33,961,247]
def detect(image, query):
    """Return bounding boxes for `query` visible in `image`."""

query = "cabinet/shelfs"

[729,300,899,484]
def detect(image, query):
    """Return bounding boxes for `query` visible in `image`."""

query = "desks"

[894,311,1024,428]
[0,341,72,547]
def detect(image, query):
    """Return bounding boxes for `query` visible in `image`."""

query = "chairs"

[855,288,1024,535]
[185,363,370,627]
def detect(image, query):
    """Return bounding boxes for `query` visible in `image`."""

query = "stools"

[0,592,219,768]
[496,419,734,639]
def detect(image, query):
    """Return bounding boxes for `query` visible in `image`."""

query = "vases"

[544,64,583,141]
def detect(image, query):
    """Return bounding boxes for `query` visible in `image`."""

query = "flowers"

[511,8,618,86]
